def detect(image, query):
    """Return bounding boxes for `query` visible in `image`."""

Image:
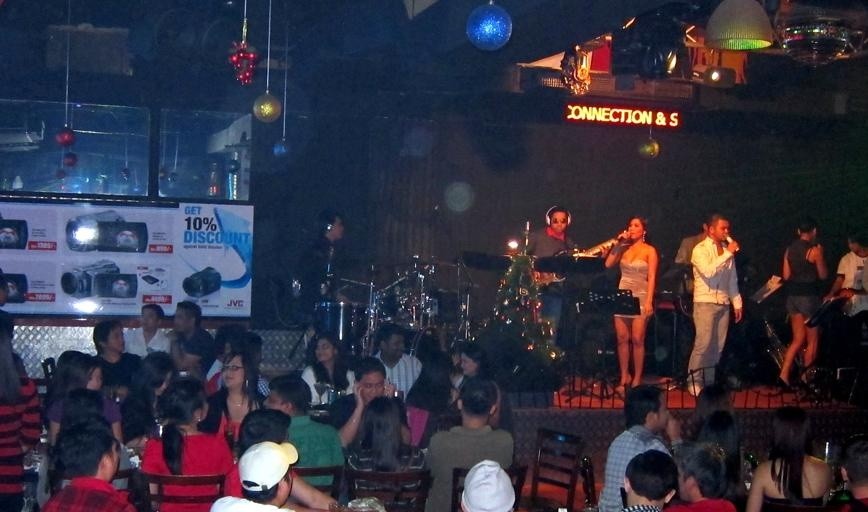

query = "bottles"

[583,486,599,511]
[839,480,851,500]
[330,383,346,404]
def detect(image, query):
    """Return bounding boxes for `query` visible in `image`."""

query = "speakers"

[481,329,567,392]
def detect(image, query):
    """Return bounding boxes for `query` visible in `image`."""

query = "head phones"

[323,223,333,233]
[545,205,572,225]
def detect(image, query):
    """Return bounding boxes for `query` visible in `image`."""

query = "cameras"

[61,260,138,299]
[65,210,148,253]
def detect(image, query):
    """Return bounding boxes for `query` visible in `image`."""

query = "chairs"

[762,502,827,511]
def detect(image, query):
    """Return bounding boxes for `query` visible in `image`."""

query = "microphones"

[616,233,630,247]
[724,233,741,253]
[524,221,530,247]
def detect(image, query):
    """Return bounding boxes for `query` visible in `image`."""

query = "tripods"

[565,314,627,404]
[449,282,475,349]
[289,243,343,361]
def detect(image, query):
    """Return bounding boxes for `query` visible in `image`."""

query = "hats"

[238,441,299,492]
[462,459,516,512]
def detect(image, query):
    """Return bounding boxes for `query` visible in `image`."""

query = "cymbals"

[420,260,457,267]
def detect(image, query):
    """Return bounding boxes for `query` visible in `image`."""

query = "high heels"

[797,379,808,394]
[775,377,799,394]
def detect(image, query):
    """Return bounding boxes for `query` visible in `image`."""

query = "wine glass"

[314,383,328,406]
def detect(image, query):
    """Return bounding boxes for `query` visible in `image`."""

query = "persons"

[687,216,745,396]
[1,267,15,341]
[773,217,829,391]
[602,217,660,388]
[1,316,40,512]
[521,206,576,363]
[826,229,868,363]
[45,301,525,512]
[600,384,831,511]
[833,433,868,512]
[673,218,713,388]
[296,207,359,307]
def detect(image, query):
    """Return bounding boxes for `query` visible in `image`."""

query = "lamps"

[54,0,78,180]
[252,1,284,124]
[122,133,131,181]
[271,49,290,158]
[467,1,514,55]
[772,25,852,73]
[167,135,181,184]
[702,1,776,51]
[639,126,662,163]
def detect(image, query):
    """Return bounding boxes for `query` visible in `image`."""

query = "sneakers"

[688,382,704,397]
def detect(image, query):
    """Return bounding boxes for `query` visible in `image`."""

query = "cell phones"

[384,375,389,397]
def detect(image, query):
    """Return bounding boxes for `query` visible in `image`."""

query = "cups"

[394,391,404,401]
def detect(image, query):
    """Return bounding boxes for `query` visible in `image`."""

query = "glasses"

[221,365,245,372]
[552,217,568,224]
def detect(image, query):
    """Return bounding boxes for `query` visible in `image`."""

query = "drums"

[314,301,369,346]
[420,289,457,324]
